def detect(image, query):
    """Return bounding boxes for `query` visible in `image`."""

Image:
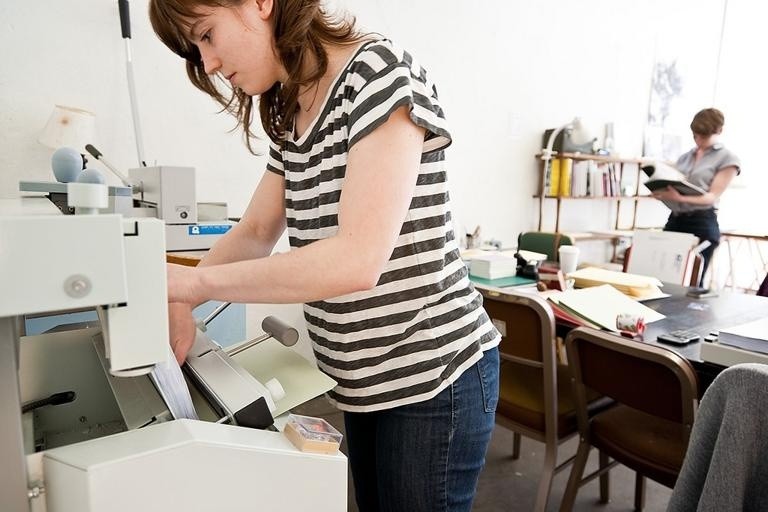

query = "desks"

[717,229,768,292]
[467,267,768,403]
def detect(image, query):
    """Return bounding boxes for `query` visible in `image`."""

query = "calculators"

[656,329,701,347]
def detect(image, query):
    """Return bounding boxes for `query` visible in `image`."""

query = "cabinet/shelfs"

[532,152,648,273]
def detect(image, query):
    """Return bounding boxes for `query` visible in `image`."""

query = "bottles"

[604,122,616,156]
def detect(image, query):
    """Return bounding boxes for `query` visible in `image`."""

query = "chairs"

[558,325,700,512]
[473,285,611,511]
[517,230,572,265]
[622,229,711,286]
[704,362,768,512]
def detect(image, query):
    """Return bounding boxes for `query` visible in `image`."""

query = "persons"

[659,105,744,288]
[143,0,505,512]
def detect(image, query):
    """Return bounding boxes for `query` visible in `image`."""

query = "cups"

[558,244,579,274]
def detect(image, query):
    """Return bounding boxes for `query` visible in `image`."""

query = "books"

[467,247,669,336]
[698,334,768,372]
[717,315,768,353]
[640,161,719,213]
[548,158,621,198]
